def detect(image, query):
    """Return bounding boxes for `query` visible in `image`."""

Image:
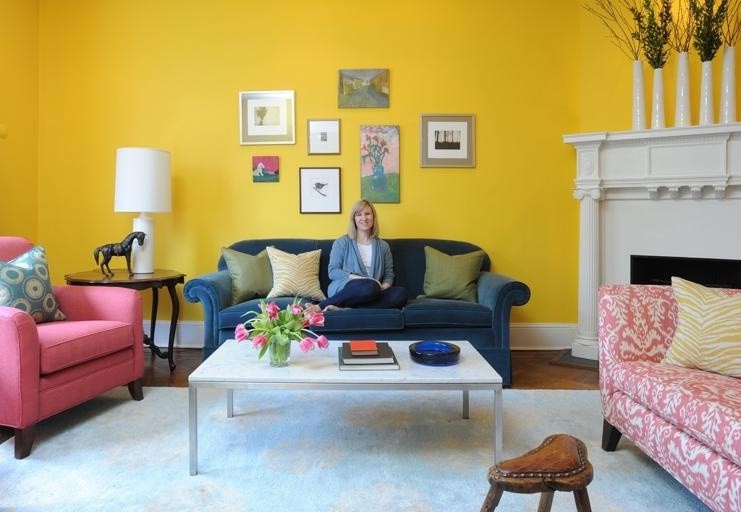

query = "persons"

[297,199,411,311]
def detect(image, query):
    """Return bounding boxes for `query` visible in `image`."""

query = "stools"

[476,433,593,512]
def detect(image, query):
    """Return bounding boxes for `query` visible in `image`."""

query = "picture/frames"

[305,118,341,156]
[297,166,341,215]
[237,91,296,147]
[419,113,477,170]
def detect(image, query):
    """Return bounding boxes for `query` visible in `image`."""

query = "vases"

[368,165,387,193]
[264,331,290,367]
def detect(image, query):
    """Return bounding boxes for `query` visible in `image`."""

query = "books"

[350,339,379,355]
[342,342,395,364]
[338,346,401,371]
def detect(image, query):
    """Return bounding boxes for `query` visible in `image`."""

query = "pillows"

[218,244,274,304]
[0,246,67,323]
[266,246,326,303]
[662,273,740,380]
[417,244,484,303]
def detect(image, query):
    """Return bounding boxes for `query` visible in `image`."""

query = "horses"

[93,231,145,278]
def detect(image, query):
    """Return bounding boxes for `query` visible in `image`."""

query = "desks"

[62,267,184,373]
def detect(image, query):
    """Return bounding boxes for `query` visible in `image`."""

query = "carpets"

[0,385,712,512]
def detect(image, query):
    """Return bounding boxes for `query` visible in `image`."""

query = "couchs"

[181,236,529,391]
[0,236,145,459]
[594,284,740,512]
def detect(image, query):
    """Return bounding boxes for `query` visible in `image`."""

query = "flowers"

[359,133,389,165]
[226,290,328,361]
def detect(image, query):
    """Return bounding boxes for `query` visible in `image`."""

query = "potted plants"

[689,0,724,125]
[665,0,696,127]
[583,0,646,130]
[629,0,670,128]
[710,0,741,126]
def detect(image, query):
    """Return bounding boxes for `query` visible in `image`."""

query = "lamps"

[112,145,174,277]
